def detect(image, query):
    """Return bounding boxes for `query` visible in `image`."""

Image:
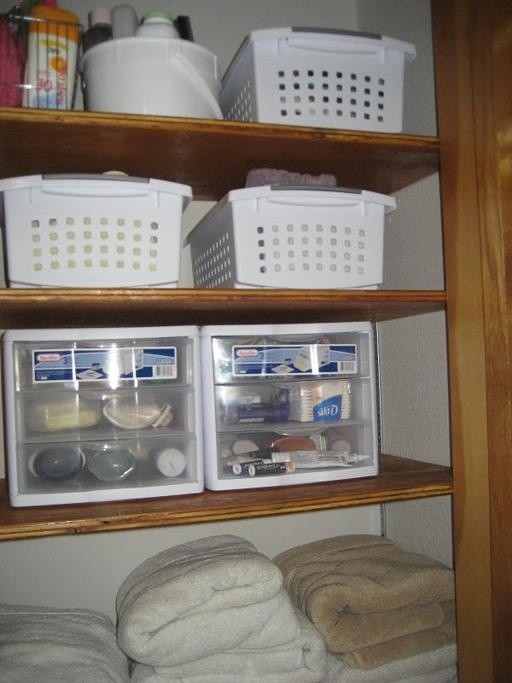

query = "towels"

[3,600,129,681]
[113,534,329,683]
[272,536,462,683]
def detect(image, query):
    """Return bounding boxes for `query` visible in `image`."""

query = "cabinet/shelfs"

[0,102,455,543]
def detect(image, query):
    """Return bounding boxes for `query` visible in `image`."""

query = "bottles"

[135,10,181,44]
[80,8,114,53]
